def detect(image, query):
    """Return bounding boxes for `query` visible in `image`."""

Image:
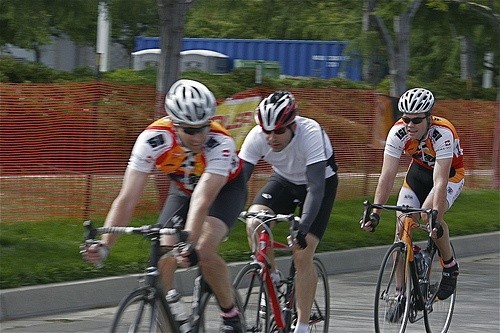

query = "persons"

[237,91,340,333]
[359,88,465,323]
[82,79,247,333]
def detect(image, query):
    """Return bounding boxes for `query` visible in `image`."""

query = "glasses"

[261,128,287,135]
[183,124,210,135]
[400,116,429,124]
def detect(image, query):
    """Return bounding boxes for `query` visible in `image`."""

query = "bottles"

[268,264,288,296]
[166,289,200,333]
[422,247,431,275]
[413,245,425,279]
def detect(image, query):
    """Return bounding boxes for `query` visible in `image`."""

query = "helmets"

[396,87,436,114]
[253,90,298,129]
[164,79,217,126]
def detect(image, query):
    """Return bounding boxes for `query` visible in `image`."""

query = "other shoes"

[385,293,408,322]
[436,262,460,300]
[256,270,284,319]
[220,311,243,333]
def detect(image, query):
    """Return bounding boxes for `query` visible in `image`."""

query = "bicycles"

[359,200,459,333]
[79,219,247,333]
[229,210,330,333]
